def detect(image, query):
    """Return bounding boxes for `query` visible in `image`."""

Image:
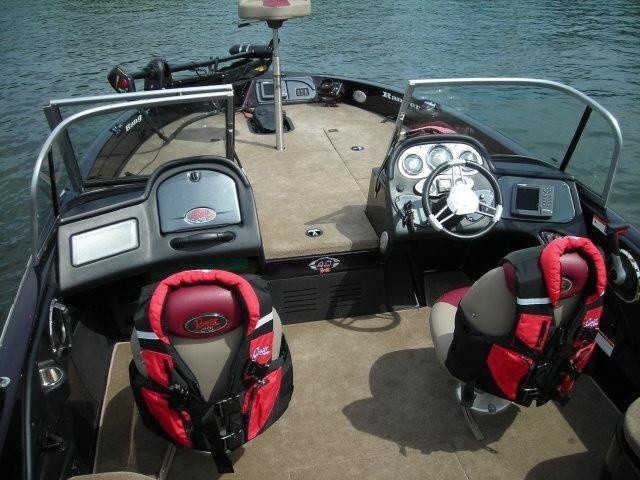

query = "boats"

[0,37,640,479]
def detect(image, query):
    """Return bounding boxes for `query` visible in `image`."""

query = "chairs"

[430,246,608,442]
[129,273,293,480]
